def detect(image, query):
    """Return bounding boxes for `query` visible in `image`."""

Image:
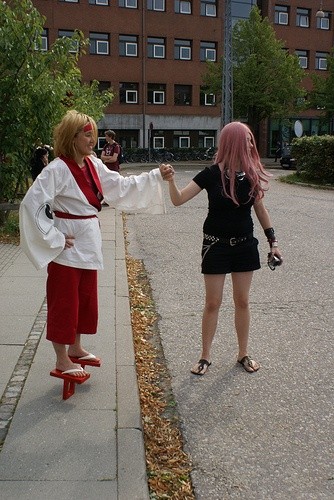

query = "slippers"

[237,356,260,372]
[50,366,90,400]
[191,359,211,376]
[67,353,101,369]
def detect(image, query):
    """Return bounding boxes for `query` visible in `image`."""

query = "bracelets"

[270,242,278,247]
[264,227,275,239]
[269,240,278,243]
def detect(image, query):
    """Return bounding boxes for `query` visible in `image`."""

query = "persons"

[30,147,49,181]
[164,122,284,374]
[98,129,120,207]
[17,109,175,378]
[274,140,282,162]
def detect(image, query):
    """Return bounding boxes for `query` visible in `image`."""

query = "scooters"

[198,146,218,161]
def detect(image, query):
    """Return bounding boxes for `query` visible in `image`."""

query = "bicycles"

[153,147,175,164]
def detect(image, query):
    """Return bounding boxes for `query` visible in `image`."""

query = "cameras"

[267,252,280,266]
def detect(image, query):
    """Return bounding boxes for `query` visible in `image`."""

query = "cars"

[279,152,305,170]
